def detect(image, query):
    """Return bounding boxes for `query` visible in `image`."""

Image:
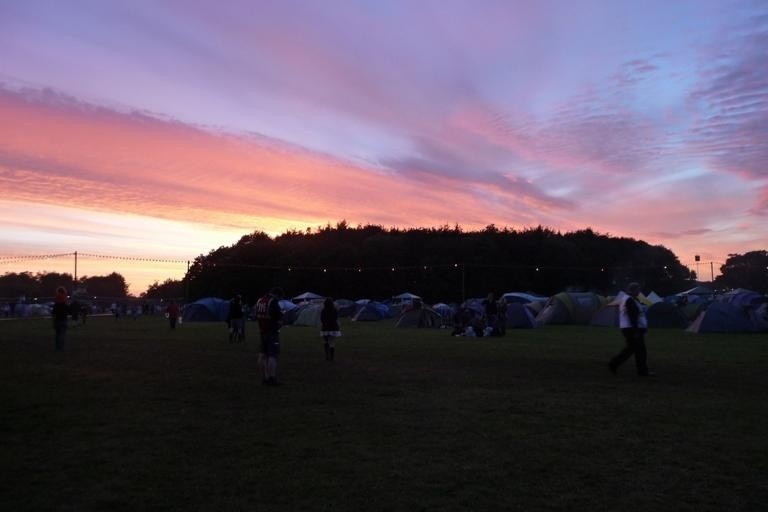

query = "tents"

[499,288,766,333]
[182,293,455,329]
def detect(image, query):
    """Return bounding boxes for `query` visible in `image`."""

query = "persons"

[451,293,513,334]
[51,287,70,352]
[255,282,284,387]
[608,282,656,376]
[4,300,162,328]
[226,296,242,344]
[166,300,180,328]
[320,297,341,361]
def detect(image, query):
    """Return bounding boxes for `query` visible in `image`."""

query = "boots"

[325,343,334,361]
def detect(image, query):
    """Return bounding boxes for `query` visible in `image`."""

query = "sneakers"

[648,369,657,376]
